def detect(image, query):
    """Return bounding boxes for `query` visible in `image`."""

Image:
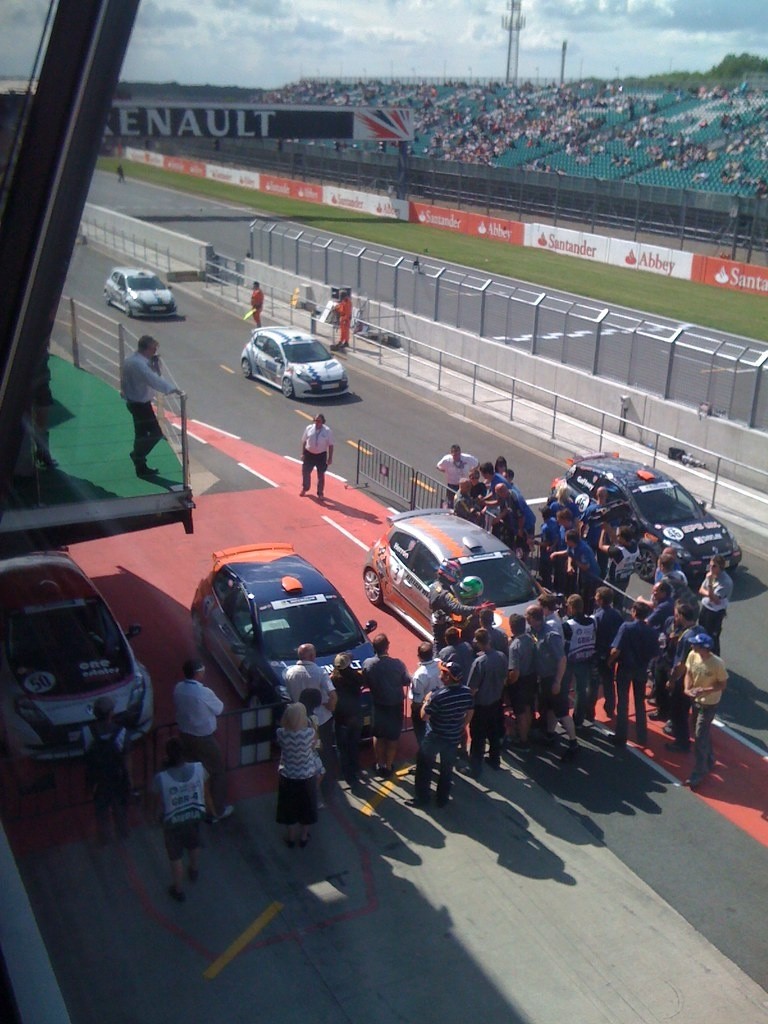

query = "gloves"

[476,600,495,616]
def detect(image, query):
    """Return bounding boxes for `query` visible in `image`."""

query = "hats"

[687,633,714,649]
[438,661,464,681]
[94,697,114,716]
[334,652,353,670]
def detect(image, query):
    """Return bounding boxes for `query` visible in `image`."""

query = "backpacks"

[83,720,131,801]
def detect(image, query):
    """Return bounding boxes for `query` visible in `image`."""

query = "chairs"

[303,83,768,199]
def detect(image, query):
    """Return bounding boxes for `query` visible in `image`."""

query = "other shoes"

[485,756,501,770]
[374,762,385,776]
[300,488,309,496]
[284,839,295,848]
[129,451,138,464]
[505,691,707,787]
[317,492,323,499]
[386,764,394,776]
[168,885,187,902]
[137,469,158,478]
[404,799,419,806]
[300,834,309,848]
[211,805,234,822]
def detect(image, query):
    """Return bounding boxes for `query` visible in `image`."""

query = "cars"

[545,450,742,581]
[0,549,156,762]
[102,266,177,318]
[363,509,565,649]
[190,541,377,723]
[240,325,349,399]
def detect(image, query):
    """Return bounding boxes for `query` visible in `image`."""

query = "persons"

[147,736,223,903]
[169,656,225,786]
[250,282,264,327]
[526,486,641,611]
[453,455,538,568]
[249,73,768,194]
[117,164,125,182]
[30,352,59,467]
[335,290,352,347]
[120,335,184,476]
[274,699,321,845]
[278,547,734,807]
[300,414,334,499]
[78,697,137,844]
[437,443,479,509]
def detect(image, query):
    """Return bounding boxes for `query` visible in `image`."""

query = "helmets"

[459,576,483,598]
[438,560,463,584]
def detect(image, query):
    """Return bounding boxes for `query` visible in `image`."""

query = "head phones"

[314,414,326,424]
